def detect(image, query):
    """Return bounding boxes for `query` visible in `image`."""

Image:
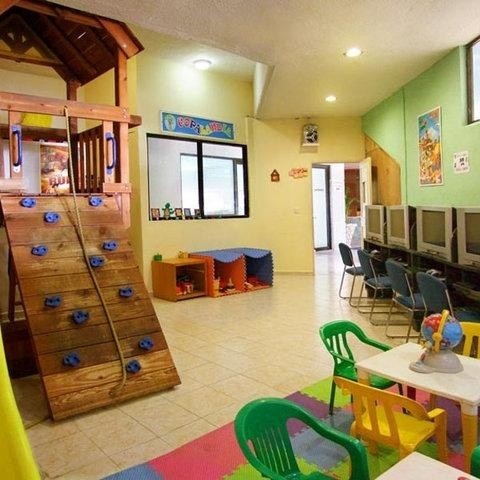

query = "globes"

[408,310,464,374]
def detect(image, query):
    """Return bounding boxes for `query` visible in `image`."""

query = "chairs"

[233,396,368,479]
[427,320,480,421]
[383,260,432,344]
[319,319,407,417]
[356,249,393,321]
[415,272,480,357]
[333,376,449,466]
[338,242,365,305]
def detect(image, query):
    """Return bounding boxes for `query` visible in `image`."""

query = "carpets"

[100,369,480,480]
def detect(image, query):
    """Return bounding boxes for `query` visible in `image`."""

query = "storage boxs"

[151,257,207,303]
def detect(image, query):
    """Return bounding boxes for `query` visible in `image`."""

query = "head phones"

[426,269,442,277]
[371,250,380,255]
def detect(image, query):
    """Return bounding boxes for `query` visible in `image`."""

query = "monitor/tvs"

[416,206,457,263]
[387,205,415,250]
[365,205,386,244]
[456,208,480,266]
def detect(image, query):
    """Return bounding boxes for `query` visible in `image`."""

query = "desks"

[354,341,480,472]
[363,239,480,324]
